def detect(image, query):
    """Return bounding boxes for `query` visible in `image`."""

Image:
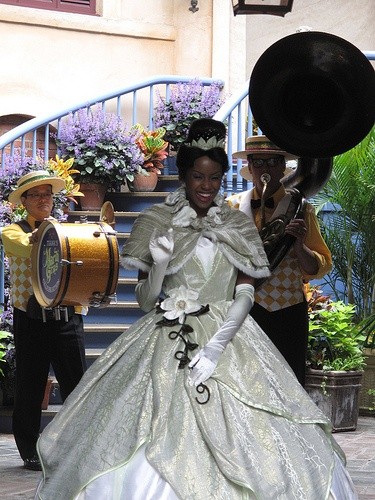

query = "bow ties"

[251,196,275,208]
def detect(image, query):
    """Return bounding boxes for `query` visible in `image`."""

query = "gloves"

[188,284,256,387]
[136,227,176,312]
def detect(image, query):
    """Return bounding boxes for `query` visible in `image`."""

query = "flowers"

[0,146,70,376]
[152,76,227,155]
[47,100,150,193]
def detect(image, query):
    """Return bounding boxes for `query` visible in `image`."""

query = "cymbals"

[100,201,115,230]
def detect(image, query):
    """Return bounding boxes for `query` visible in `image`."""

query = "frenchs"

[248,30,375,288]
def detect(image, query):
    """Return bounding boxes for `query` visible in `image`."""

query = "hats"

[232,135,300,161]
[8,170,66,206]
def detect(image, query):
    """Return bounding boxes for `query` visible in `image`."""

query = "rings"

[302,227,306,231]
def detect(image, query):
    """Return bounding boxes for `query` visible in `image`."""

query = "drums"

[31,219,121,315]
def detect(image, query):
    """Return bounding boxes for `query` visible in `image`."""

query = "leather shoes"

[23,457,44,472]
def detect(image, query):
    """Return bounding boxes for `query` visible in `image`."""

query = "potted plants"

[305,301,369,434]
[125,124,169,191]
[342,313,375,417]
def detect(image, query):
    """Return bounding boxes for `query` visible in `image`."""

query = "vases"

[165,156,179,175]
[74,183,106,210]
[48,153,85,214]
[41,380,53,410]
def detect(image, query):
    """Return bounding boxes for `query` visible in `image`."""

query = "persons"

[0,169,88,471]
[37,118,360,500]
[225,134,333,387]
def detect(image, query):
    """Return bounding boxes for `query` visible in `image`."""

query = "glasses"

[27,192,54,199]
[250,157,282,168]
